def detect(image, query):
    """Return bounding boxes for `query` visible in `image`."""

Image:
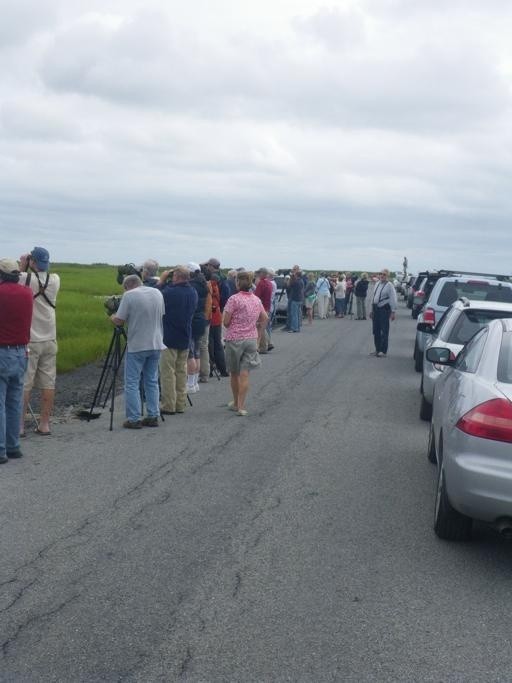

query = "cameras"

[117,263,143,284]
[103,296,122,316]
[275,269,291,276]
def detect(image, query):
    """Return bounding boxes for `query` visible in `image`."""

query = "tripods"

[270,279,288,330]
[88,325,165,431]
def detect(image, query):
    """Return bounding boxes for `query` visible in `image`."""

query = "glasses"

[380,273,387,276]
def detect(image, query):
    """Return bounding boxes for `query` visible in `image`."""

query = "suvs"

[412,277,512,370]
[420,298,512,421]
[401,272,460,319]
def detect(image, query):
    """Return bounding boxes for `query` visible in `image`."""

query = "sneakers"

[7,450,23,458]
[238,410,247,416]
[377,351,386,357]
[123,420,143,429]
[0,457,8,463]
[369,351,378,356]
[143,417,159,426]
[195,385,199,391]
[159,409,174,415]
[229,401,240,411]
[184,386,196,394]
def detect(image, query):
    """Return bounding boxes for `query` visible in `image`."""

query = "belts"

[0,344,25,350]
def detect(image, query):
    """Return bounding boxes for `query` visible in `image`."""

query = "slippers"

[34,426,52,436]
[18,431,27,437]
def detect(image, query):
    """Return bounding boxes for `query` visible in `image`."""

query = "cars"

[273,275,290,319]
[426,318,512,541]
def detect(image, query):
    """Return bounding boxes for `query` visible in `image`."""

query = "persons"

[208,258,228,312]
[290,270,304,332]
[403,256,407,278]
[305,272,315,325]
[138,260,161,401]
[331,275,346,317]
[18,247,61,437]
[227,269,237,292]
[183,262,208,394]
[223,271,268,416]
[198,264,212,383]
[282,264,300,330]
[343,269,358,304]
[355,272,369,319]
[110,275,166,429]
[254,268,272,353]
[201,267,228,377]
[153,265,197,415]
[329,269,337,312]
[267,268,276,348]
[315,271,330,319]
[0,258,33,464]
[368,269,396,357]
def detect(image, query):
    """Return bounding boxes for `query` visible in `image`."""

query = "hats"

[206,259,220,267]
[255,267,267,274]
[187,263,201,273]
[30,247,50,270]
[266,267,274,274]
[0,258,20,275]
